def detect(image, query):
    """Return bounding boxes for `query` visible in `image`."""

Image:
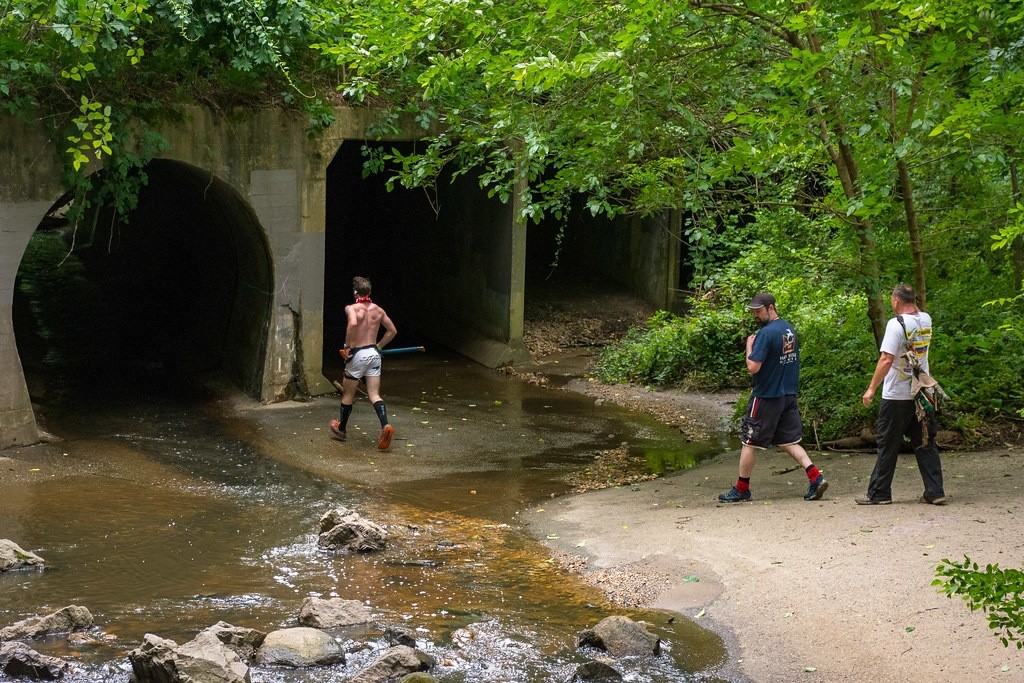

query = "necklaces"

[773,316,778,319]
[356,297,372,303]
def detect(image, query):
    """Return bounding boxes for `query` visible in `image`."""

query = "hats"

[746,294,776,309]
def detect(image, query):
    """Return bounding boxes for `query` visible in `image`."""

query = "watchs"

[344,344,349,348]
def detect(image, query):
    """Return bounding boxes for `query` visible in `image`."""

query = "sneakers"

[377,424,393,451]
[919,495,946,504]
[804,474,828,501]
[718,487,751,503]
[330,419,346,440]
[855,493,892,505]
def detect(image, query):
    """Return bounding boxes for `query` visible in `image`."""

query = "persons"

[854,283,946,506]
[718,293,828,501]
[330,277,397,449]
